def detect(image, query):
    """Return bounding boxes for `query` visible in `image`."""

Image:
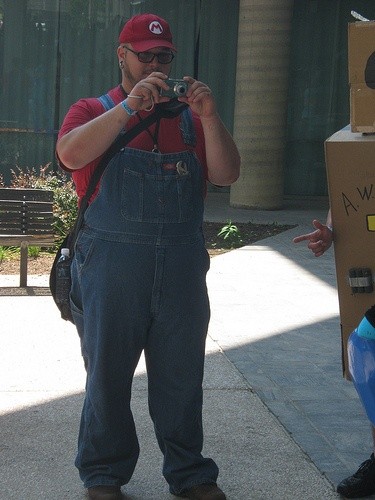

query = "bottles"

[55,248,71,303]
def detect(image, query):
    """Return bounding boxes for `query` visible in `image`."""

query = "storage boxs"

[348,20,375,134]
[323,124,375,381]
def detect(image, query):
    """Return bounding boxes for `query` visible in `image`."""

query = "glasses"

[122,45,175,64]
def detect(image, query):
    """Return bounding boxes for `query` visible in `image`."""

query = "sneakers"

[337,452,375,498]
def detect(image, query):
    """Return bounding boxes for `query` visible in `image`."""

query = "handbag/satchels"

[50,230,76,326]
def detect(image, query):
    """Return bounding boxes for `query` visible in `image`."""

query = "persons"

[293,208,335,256]
[56,13,241,500]
[336,305,375,500]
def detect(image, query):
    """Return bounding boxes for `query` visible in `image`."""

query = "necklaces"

[119,81,162,158]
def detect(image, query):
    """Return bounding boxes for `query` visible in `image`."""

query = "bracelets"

[121,100,134,115]
[327,224,333,232]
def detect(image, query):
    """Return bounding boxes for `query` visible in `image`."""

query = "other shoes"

[88,485,122,500]
[180,482,226,500]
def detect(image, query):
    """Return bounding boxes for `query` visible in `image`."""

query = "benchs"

[0,187,59,296]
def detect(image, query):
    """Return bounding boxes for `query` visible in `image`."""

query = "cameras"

[161,79,189,98]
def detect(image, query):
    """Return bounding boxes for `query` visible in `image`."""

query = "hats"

[119,13,178,53]
